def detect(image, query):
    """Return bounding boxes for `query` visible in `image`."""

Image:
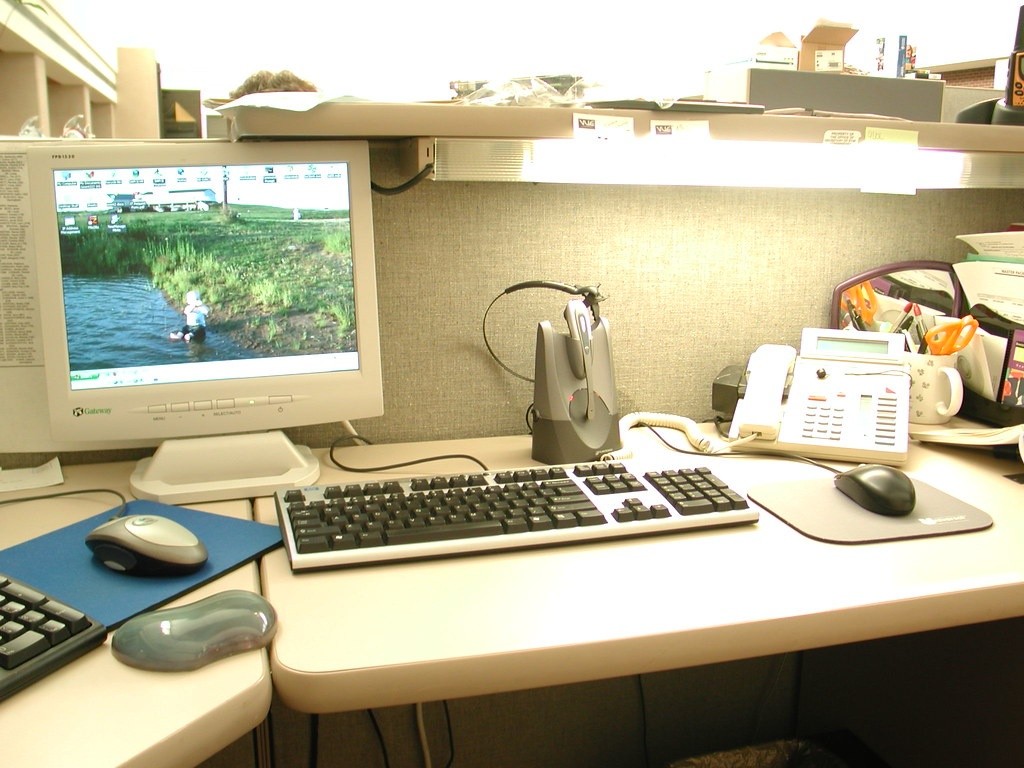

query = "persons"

[169,290,209,340]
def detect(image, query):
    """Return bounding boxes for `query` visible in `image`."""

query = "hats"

[187,291,200,303]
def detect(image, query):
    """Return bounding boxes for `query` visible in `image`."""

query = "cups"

[901,344,965,425]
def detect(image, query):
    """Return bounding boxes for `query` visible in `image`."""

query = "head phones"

[484,281,603,383]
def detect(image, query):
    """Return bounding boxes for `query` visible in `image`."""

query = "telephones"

[954,4,1024,126]
[724,326,913,467]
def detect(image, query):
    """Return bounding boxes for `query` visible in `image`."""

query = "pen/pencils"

[897,301,932,354]
[846,296,914,339]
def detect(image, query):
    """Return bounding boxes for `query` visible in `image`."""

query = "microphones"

[577,311,596,420]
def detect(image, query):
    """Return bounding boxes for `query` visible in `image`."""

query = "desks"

[258,405,1024,719]
[0,457,276,768]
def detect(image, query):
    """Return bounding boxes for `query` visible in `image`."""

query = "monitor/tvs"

[28,139,384,442]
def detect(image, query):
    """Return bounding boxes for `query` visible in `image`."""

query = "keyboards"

[0,571,107,703]
[272,457,758,572]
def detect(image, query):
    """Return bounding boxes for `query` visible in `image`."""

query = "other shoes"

[170,332,181,339]
[184,334,190,344]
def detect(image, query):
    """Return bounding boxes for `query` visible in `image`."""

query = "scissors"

[926,316,979,356]
[841,279,879,326]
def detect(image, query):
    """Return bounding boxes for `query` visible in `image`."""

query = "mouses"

[836,462,916,518]
[83,515,209,577]
